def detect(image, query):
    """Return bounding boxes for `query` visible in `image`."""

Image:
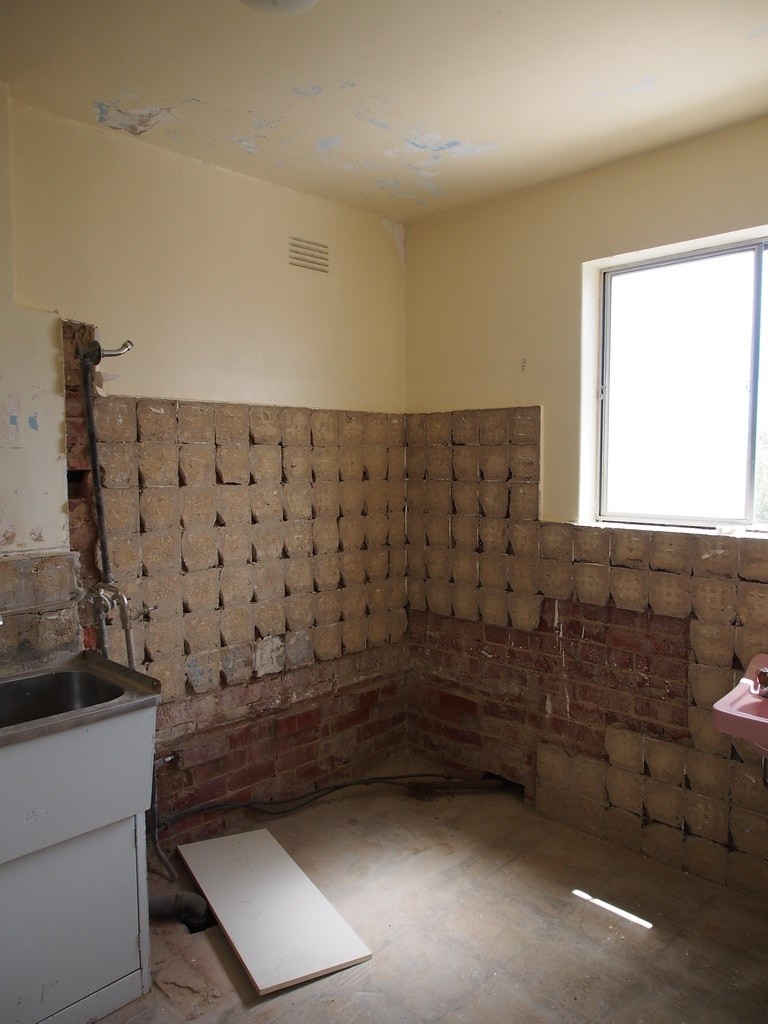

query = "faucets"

[756,667,768,699]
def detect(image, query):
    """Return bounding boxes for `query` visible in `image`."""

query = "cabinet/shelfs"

[0,648,162,1024]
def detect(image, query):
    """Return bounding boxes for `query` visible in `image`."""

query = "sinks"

[0,665,163,865]
[712,678,768,757]
